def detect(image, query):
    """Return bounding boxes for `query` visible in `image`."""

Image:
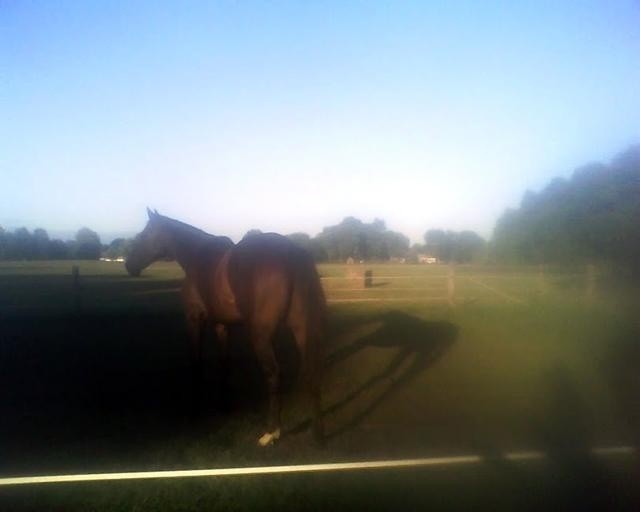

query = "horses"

[124,205,330,447]
